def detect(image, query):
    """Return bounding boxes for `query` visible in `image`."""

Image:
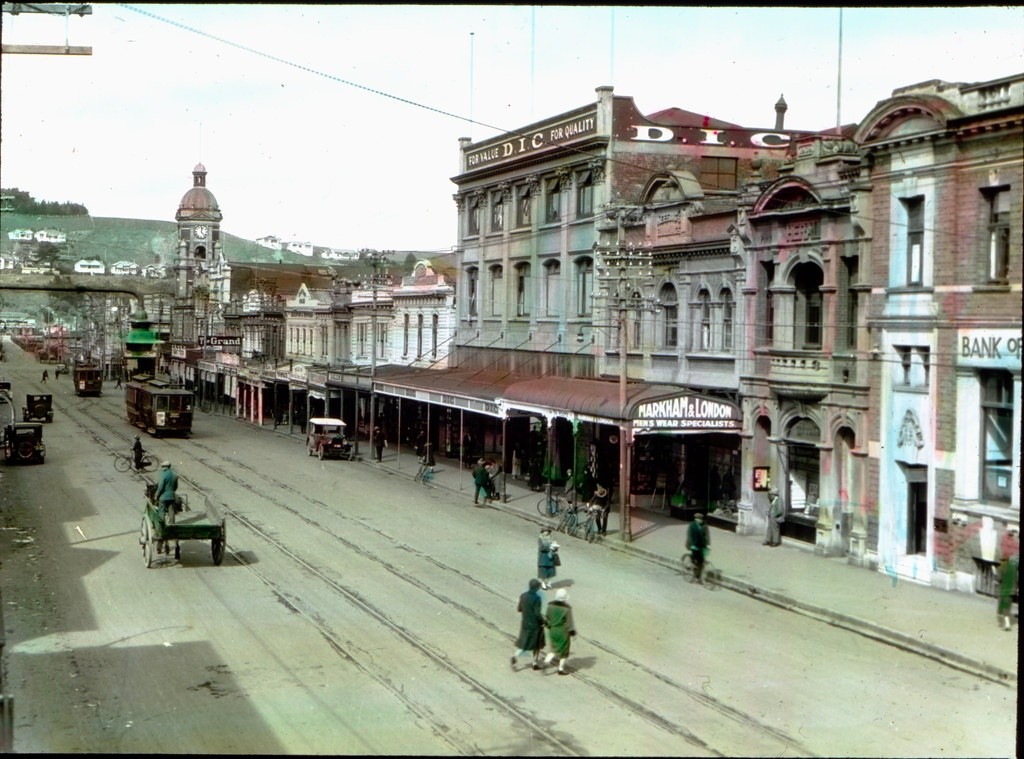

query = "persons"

[538,527,558,589]
[132,435,143,472]
[580,467,595,509]
[542,588,577,675]
[762,488,783,547]
[565,469,573,508]
[114,377,123,390]
[530,462,539,491]
[586,481,611,537]
[511,579,546,671]
[992,554,1018,631]
[282,411,288,424]
[686,512,711,581]
[374,426,388,463]
[40,369,49,383]
[513,448,521,482]
[155,462,178,524]
[55,368,60,380]
[414,431,426,463]
[464,432,474,468]
[472,458,493,503]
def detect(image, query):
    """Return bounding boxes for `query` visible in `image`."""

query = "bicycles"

[681,549,717,590]
[113,448,161,472]
[538,491,602,543]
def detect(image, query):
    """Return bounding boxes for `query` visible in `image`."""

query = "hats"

[694,513,704,520]
[540,526,552,534]
[555,588,569,601]
[161,460,171,467]
[477,457,485,464]
[769,488,780,495]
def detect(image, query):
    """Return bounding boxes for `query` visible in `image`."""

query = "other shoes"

[771,541,781,547]
[512,656,516,665]
[763,541,772,545]
[533,664,541,670]
[546,581,551,588]
[558,669,568,675]
[542,582,546,590]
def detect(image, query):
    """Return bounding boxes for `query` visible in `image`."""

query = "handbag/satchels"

[553,554,561,566]
[384,440,388,447]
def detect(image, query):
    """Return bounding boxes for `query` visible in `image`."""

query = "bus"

[74,367,103,396]
[125,380,194,437]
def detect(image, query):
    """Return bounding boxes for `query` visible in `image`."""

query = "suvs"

[23,393,54,423]
[1,423,46,464]
[307,418,354,460]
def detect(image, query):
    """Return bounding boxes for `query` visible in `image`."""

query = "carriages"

[139,482,227,568]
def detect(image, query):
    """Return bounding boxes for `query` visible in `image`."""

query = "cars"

[57,364,69,374]
[0,382,12,403]
